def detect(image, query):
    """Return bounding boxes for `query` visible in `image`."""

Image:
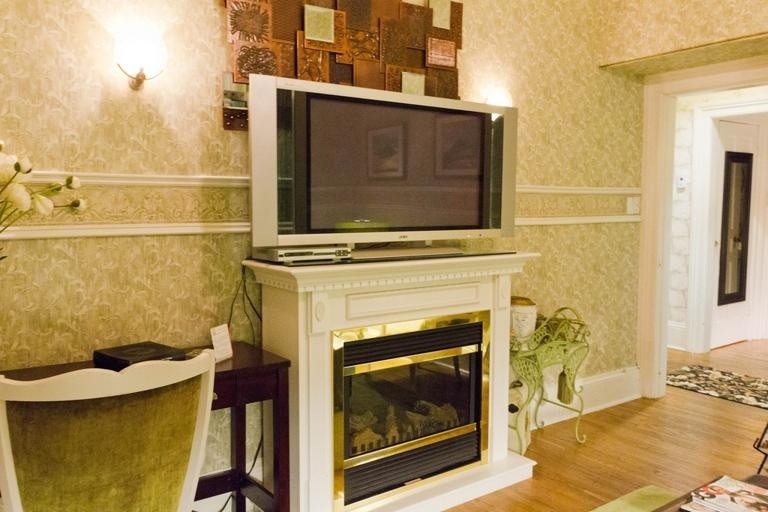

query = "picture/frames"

[364,115,484,184]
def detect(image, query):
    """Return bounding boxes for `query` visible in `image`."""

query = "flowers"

[0,143,88,261]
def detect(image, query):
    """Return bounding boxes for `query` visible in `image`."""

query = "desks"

[1,338,292,511]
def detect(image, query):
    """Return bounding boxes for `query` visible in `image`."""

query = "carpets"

[664,363,768,410]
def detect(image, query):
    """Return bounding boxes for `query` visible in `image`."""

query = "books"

[679,474,768,512]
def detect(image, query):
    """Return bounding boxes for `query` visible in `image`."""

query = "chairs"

[1,346,217,512]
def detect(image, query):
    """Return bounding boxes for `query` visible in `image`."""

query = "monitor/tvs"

[249,72,521,263]
